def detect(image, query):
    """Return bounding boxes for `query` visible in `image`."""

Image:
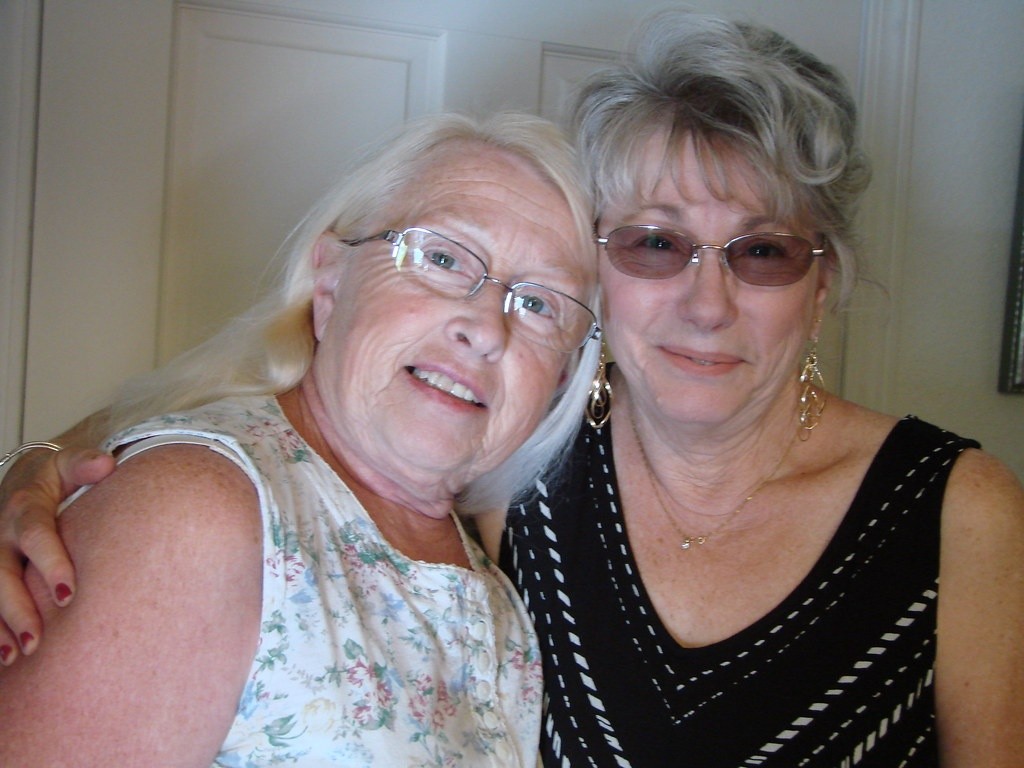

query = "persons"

[0,3,1024,768]
[0,109,602,768]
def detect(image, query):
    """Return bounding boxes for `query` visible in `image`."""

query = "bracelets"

[0,441,64,468]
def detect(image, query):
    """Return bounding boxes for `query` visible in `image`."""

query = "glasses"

[592,224,827,288]
[339,226,602,353]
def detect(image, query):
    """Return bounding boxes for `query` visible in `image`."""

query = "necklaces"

[630,418,797,549]
